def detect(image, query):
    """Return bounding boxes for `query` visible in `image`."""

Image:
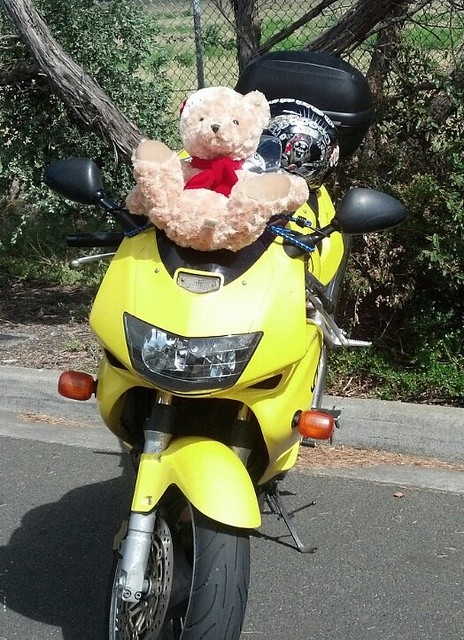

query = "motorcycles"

[45,50,410,640]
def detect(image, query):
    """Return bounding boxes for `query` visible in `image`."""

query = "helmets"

[257,97,339,192]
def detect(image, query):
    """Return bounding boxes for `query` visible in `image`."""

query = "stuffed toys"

[126,87,309,256]
